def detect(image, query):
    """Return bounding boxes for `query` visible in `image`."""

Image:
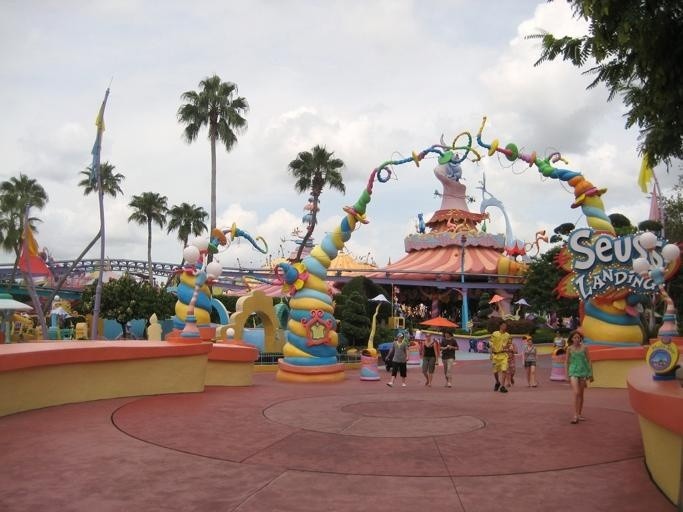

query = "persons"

[552,329,565,348]
[385,332,409,388]
[563,332,594,424]
[523,338,537,386]
[438,328,459,388]
[488,321,512,392]
[418,333,439,389]
[506,342,518,387]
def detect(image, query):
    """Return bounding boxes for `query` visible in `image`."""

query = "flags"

[637,153,653,194]
[648,183,659,222]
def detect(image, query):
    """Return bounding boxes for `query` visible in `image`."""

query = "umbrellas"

[416,314,459,329]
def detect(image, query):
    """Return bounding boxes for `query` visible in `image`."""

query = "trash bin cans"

[549,348,568,381]
[359,348,380,381]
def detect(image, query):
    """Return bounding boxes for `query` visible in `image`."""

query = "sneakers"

[425,380,431,386]
[500,386,508,392]
[571,414,585,423]
[494,382,500,391]
[402,383,406,387]
[528,383,536,387]
[444,382,452,387]
[387,381,393,387]
[507,379,514,387]
